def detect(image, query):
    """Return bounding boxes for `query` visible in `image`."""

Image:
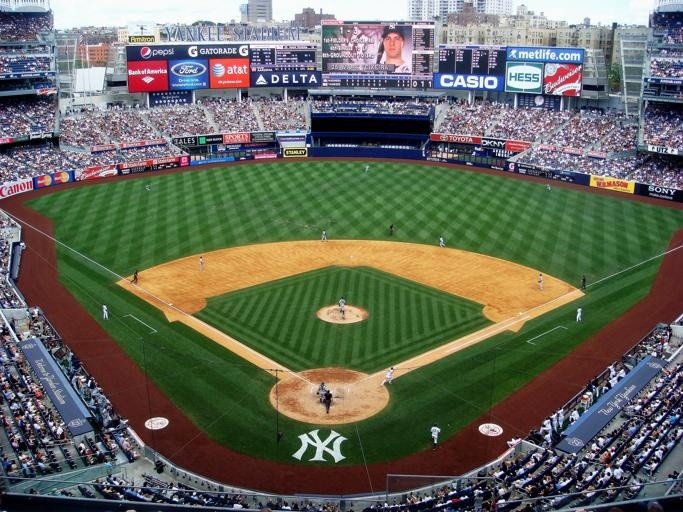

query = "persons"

[0,13,683,512]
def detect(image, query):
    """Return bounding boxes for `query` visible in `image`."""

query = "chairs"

[0,304,682,511]
